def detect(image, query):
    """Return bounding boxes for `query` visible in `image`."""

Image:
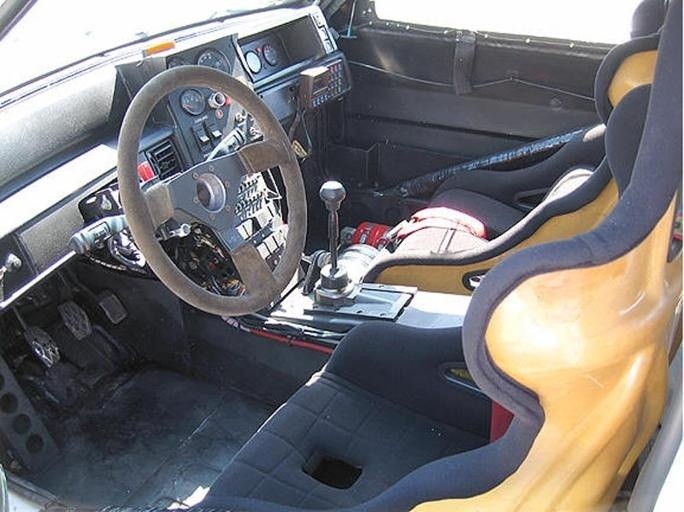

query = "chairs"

[184,0,683,512]
[361,0,683,296]
